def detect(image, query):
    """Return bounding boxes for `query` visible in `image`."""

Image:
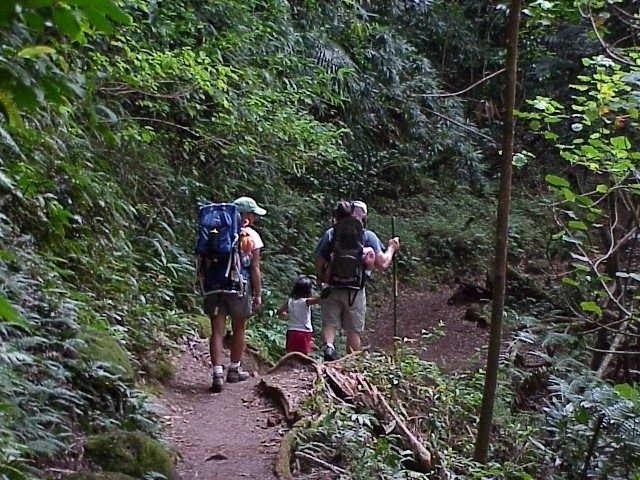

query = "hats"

[232,196,268,216]
[352,200,368,214]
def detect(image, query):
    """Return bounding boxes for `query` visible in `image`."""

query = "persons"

[197,196,267,393]
[313,200,400,361]
[277,277,321,371]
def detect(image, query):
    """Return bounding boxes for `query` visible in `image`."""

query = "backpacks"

[193,201,246,297]
[328,201,365,291]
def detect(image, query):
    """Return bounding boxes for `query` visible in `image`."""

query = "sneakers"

[227,367,247,383]
[213,372,225,392]
[325,345,336,361]
